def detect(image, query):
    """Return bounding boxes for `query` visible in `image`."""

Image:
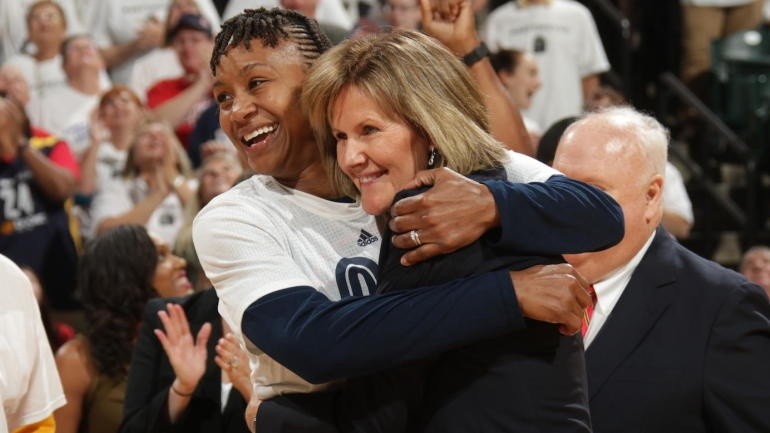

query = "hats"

[168,14,212,36]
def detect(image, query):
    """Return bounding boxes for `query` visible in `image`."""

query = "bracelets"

[460,41,489,67]
[172,385,193,396]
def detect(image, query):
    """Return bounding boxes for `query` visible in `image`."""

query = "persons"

[0,0,418,307]
[0,254,67,433]
[547,105,770,433]
[419,0,694,241]
[192,7,625,401]
[54,222,195,433]
[122,286,249,433]
[245,29,594,433]
[739,244,770,294]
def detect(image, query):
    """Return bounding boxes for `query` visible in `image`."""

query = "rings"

[411,230,421,245]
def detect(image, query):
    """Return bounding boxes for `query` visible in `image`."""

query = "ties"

[580,285,598,340]
